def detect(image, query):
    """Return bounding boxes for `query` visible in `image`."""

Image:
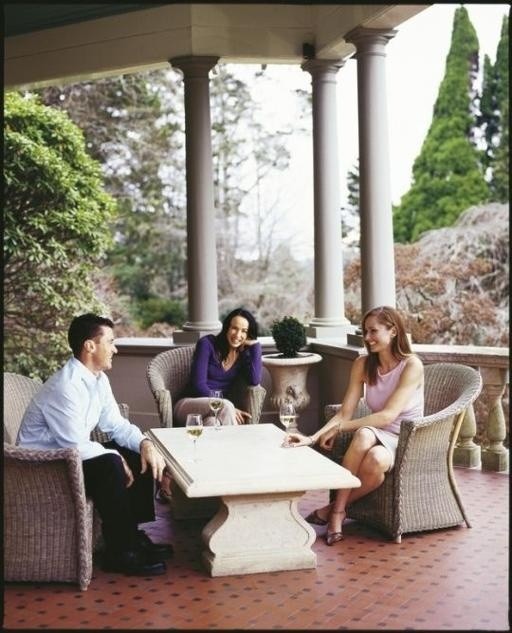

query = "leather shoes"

[102,531,174,574]
[160,478,172,503]
[326,509,347,546]
[305,510,327,524]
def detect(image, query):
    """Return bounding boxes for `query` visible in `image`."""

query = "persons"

[170,306,264,426]
[14,311,175,576]
[277,304,426,544]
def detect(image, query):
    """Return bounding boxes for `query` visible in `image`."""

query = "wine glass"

[183,412,205,461]
[279,401,299,449]
[208,389,225,431]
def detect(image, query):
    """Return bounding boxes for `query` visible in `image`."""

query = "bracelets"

[338,424,341,434]
[309,435,314,447]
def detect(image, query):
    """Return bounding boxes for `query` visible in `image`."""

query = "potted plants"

[258,314,323,414]
